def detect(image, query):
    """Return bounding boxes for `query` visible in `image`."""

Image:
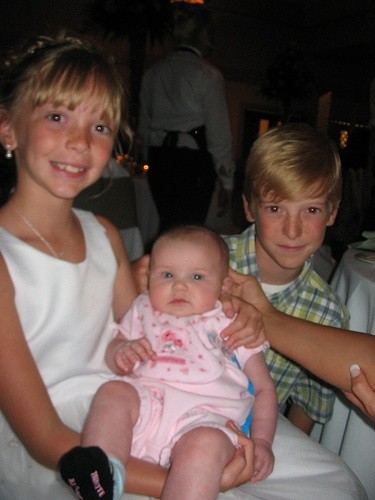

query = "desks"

[131,176,226,248]
[310,243,375,500]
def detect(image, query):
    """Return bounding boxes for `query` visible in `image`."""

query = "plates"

[353,253,375,264]
[348,241,375,253]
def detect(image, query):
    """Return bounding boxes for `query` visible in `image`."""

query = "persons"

[58,223,277,499]
[133,5,236,235]
[218,125,351,435]
[221,268,375,426]
[1,42,366,500]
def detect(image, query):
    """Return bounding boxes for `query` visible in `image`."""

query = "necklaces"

[10,204,73,259]
